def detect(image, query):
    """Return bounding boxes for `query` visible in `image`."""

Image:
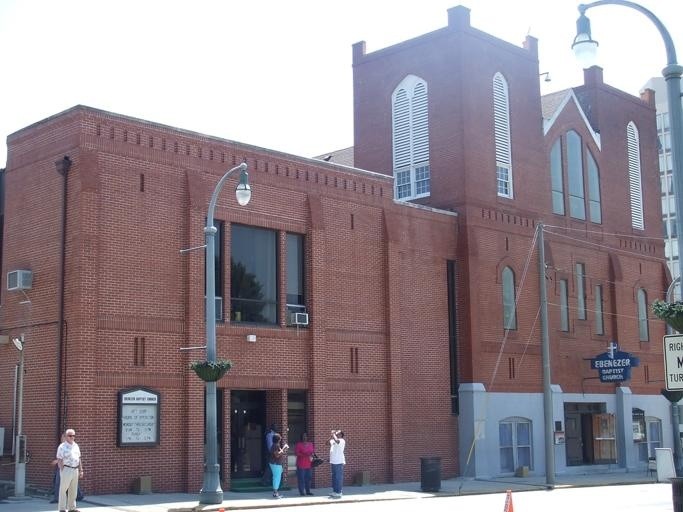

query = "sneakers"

[329,491,342,497]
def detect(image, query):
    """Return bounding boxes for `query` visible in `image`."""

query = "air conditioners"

[291,312,309,326]
[7,270,32,290]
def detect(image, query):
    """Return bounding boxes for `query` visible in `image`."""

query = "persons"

[55,427,83,512]
[294,430,314,496]
[263,424,277,485]
[267,433,289,497]
[244,421,261,472]
[48,432,83,504]
[324,429,346,497]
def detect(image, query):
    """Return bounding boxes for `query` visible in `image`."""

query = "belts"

[65,465,78,469]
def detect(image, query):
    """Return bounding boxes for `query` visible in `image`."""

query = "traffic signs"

[663,334,682,392]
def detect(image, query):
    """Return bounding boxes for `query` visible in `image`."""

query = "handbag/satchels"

[311,458,323,467]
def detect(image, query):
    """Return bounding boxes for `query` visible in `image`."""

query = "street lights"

[12,337,27,496]
[571,0,683,300]
[199,162,251,504]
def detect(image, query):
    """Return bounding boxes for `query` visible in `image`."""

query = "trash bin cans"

[418,455,442,491]
[666,476,683,512]
